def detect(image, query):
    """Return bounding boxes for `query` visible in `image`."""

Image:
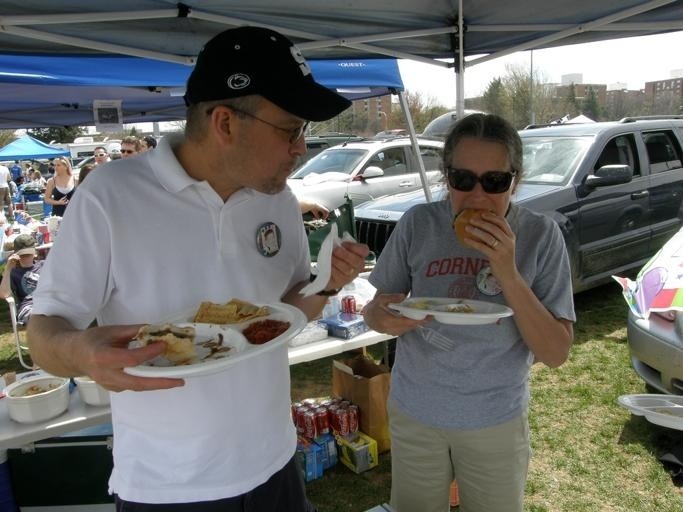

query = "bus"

[0,159,49,173]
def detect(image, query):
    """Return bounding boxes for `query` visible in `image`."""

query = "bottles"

[31,211,53,246]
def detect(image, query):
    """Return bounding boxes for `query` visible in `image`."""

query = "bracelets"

[308,273,342,297]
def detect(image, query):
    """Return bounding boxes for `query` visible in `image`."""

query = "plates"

[122,303,307,378]
[388,297,513,327]
[614,393,683,429]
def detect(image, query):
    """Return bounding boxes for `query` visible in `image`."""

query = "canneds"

[341,295,356,314]
[291,396,360,439]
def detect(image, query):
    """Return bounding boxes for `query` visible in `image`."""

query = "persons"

[361,111,579,511]
[24,25,355,511]
[295,198,328,222]
[0,134,156,328]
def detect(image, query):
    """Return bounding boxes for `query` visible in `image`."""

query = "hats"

[14,234,36,256]
[184,26,353,122]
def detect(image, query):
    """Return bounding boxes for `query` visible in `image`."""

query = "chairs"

[3,298,41,371]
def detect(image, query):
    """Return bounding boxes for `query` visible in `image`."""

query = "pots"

[74,372,110,408]
[4,375,72,423]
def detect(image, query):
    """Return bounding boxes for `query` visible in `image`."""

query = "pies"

[132,324,196,365]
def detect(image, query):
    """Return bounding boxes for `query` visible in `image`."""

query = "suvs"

[282,136,450,231]
[301,135,365,167]
[349,115,683,299]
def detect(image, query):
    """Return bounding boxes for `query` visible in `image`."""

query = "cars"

[626,225,683,394]
[375,129,408,139]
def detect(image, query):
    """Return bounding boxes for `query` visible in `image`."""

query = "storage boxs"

[12,199,48,221]
[336,430,380,473]
[296,431,323,483]
[316,433,338,469]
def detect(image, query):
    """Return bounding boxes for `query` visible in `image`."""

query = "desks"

[0,242,52,264]
[0,278,397,464]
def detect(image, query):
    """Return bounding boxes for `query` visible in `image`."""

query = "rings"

[491,240,498,249]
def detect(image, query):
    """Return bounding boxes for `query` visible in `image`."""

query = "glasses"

[206,102,310,147]
[445,163,518,194]
[119,148,134,155]
[94,153,107,158]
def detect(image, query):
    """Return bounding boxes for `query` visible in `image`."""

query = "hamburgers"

[451,208,497,247]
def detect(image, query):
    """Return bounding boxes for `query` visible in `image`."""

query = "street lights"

[377,110,387,130]
